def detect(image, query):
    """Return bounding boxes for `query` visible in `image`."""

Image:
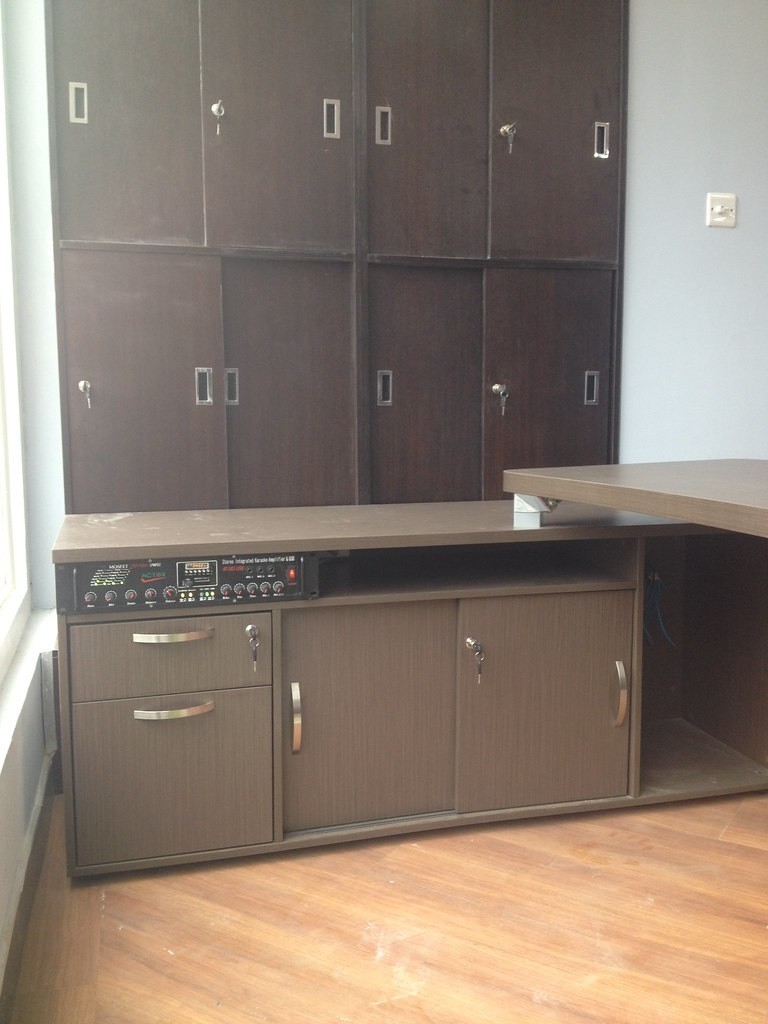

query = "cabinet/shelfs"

[278,586,639,853]
[62,605,279,878]
[61,245,364,515]
[364,260,625,507]
[41,0,359,256]
[359,0,624,266]
